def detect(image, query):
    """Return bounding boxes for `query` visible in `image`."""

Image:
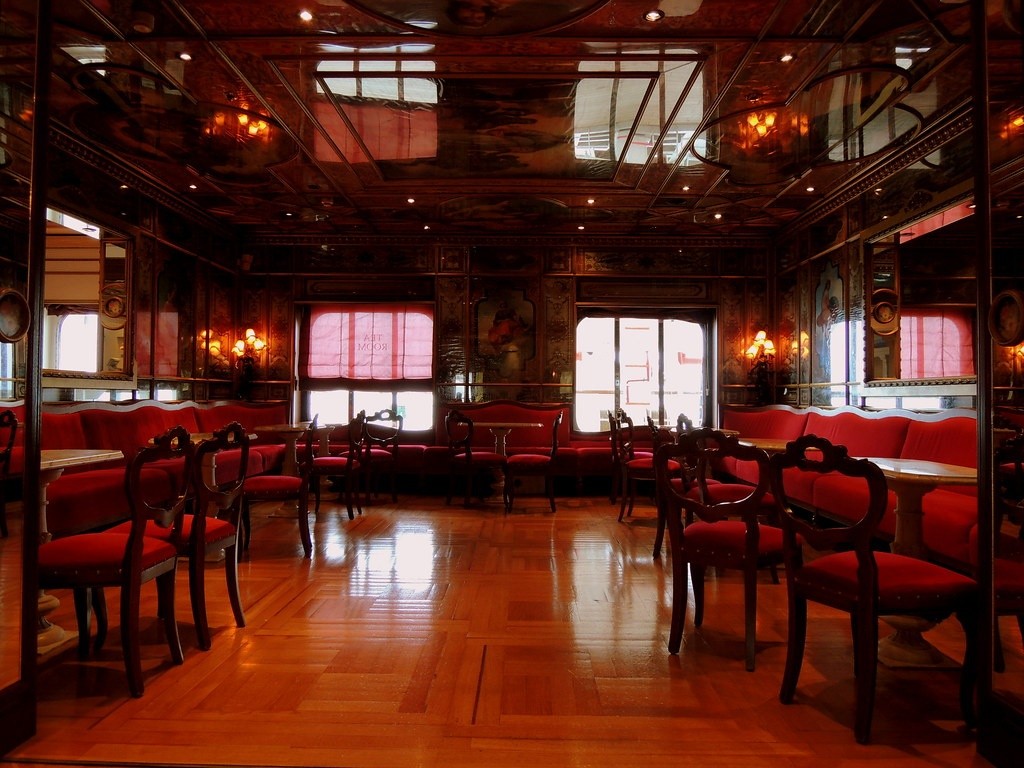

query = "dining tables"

[148,431,259,562]
[458,421,545,504]
[847,456,980,670]
[692,428,740,480]
[738,438,822,518]
[251,425,312,518]
[296,420,343,502]
[37,450,123,656]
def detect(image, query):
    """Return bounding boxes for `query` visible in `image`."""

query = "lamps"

[745,330,776,385]
[230,328,266,381]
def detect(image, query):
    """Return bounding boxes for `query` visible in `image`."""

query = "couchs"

[39,398,978,585]
[0,399,25,503]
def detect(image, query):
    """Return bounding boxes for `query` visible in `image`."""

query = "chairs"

[0,408,18,538]
[993,429,1024,674]
[37,423,198,699]
[236,414,318,563]
[444,408,510,510]
[502,409,565,513]
[87,421,252,653]
[765,432,979,746]
[300,410,366,520]
[339,409,403,507]
[607,405,810,672]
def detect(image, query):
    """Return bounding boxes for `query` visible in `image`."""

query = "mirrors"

[977,0,1024,718]
[0,0,42,692]
